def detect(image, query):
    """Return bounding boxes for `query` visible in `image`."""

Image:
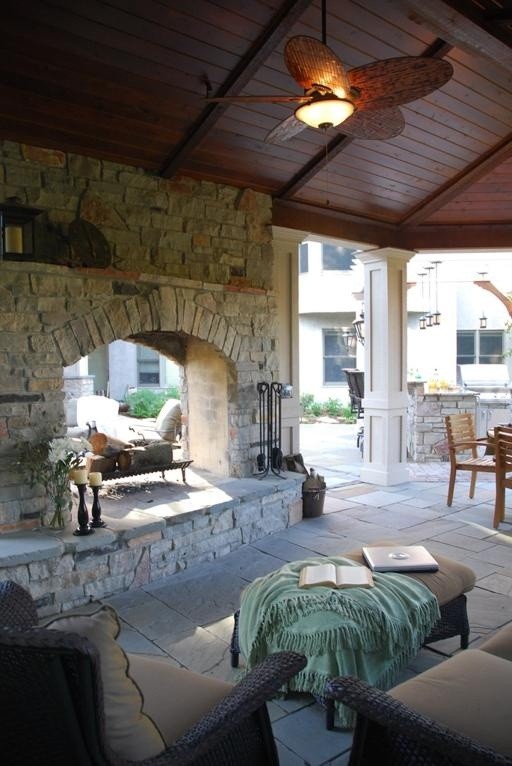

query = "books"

[299,563,377,590]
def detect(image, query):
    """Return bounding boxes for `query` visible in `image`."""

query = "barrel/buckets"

[304,484,327,518]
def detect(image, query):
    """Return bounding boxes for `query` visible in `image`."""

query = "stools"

[234,538,477,730]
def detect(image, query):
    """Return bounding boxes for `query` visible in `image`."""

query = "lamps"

[475,268,491,330]
[416,260,443,329]
[351,319,365,348]
[339,326,356,354]
[288,83,358,132]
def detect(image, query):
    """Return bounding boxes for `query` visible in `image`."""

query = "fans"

[204,1,456,146]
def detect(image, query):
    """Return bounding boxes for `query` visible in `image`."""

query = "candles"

[70,466,90,486]
[88,472,104,486]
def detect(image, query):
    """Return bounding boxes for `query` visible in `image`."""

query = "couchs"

[323,620,511,766]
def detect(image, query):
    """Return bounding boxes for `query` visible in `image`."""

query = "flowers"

[19,434,92,522]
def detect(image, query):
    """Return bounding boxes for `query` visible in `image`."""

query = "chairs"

[492,424,512,528]
[0,577,304,764]
[444,413,506,525]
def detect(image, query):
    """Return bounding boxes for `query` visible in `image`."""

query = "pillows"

[154,398,181,443]
[29,603,167,765]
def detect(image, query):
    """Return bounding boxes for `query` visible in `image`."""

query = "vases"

[38,481,74,531]
